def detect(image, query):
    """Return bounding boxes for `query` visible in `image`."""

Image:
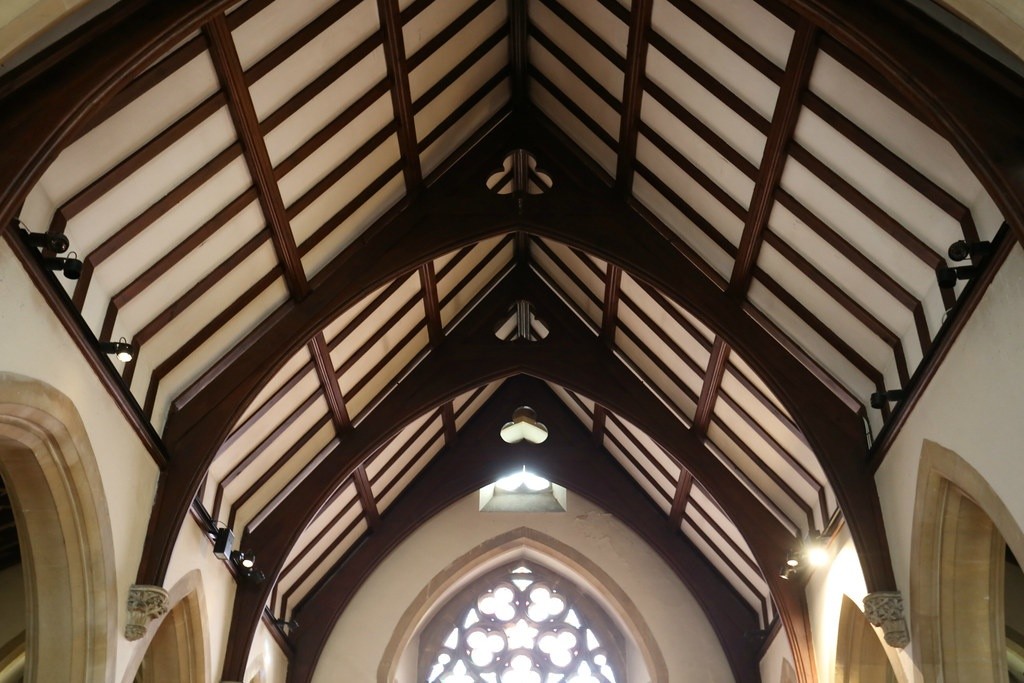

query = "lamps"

[47,257,83,280]
[212,520,235,559]
[937,236,991,287]
[231,548,255,568]
[276,618,299,632]
[871,389,904,408]
[100,341,134,362]
[779,525,825,580]
[21,230,69,254]
